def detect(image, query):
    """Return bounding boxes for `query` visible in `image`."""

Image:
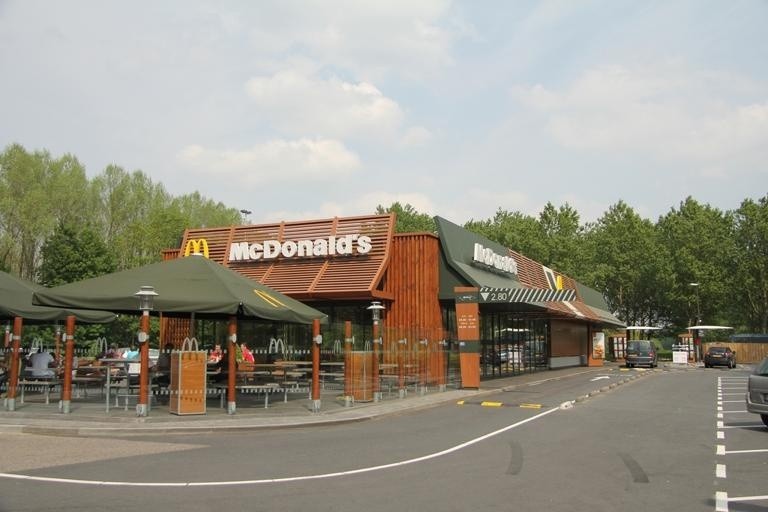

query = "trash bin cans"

[170,351,207,416]
[672,344,688,364]
[352,352,374,403]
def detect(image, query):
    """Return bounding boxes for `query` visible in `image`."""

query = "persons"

[27,344,56,388]
[104,338,255,387]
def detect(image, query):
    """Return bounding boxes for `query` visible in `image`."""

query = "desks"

[248,360,417,400]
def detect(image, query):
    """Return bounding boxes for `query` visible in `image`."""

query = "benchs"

[19,375,414,409]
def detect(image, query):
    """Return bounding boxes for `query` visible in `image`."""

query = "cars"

[704,346,737,369]
[745,351,768,426]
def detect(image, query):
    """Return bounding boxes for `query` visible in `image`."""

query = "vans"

[522,341,546,367]
[624,340,659,369]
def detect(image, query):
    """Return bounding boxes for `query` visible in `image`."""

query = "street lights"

[367,301,385,404]
[133,285,159,417]
[688,283,701,325]
